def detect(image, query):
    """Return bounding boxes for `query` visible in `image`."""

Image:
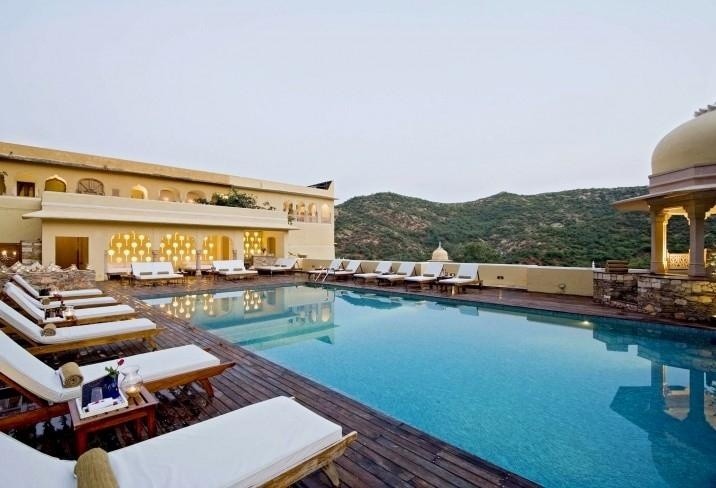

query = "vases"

[119,364,145,399]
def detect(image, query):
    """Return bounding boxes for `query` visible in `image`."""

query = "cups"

[91,387,103,409]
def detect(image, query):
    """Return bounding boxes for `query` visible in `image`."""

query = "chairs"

[0,392,362,488]
[0,300,164,358]
[0,329,244,431]
[2,272,136,323]
[102,257,484,298]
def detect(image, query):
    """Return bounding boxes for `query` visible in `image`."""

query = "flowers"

[105,358,126,374]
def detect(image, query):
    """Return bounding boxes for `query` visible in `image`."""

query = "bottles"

[121,364,143,398]
